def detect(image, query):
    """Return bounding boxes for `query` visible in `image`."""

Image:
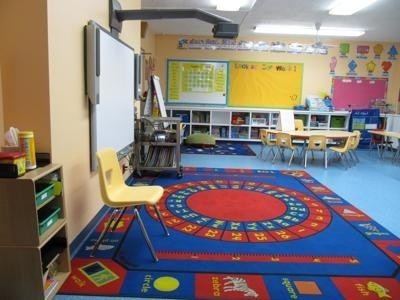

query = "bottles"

[17,131,37,171]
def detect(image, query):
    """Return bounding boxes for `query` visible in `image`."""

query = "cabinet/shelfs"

[0,163,71,300]
[350,112,393,150]
[159,106,350,145]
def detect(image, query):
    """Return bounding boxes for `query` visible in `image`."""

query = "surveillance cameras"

[250,24,258,31]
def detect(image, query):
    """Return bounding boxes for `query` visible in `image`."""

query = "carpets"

[180,141,256,156]
[57,166,400,299]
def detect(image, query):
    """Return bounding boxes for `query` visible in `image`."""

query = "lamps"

[251,23,368,37]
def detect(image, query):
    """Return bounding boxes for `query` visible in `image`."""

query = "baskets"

[330,117,345,128]
[36,181,55,205]
[39,206,62,235]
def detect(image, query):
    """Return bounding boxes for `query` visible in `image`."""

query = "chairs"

[370,128,385,154]
[90,146,171,263]
[256,119,361,169]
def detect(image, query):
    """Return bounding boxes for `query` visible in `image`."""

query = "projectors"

[212,24,240,39]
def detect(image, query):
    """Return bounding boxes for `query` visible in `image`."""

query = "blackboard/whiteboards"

[86,20,136,172]
[152,75,169,128]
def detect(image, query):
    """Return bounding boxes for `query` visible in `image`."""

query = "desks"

[367,130,400,164]
[257,130,353,166]
